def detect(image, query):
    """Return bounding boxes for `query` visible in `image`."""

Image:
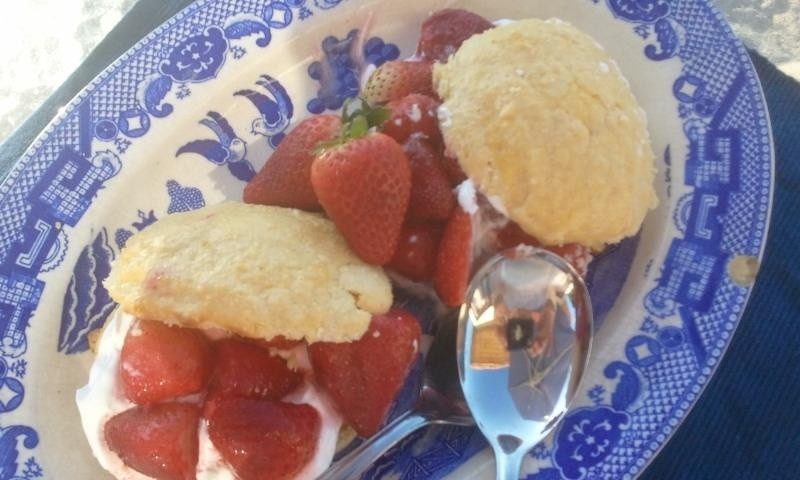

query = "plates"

[0,0,775,480]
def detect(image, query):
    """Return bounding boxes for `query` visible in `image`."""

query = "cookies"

[430,20,659,253]
[75,200,424,480]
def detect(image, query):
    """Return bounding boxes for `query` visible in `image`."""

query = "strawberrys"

[244,8,590,309]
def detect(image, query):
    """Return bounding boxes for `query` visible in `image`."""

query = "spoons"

[454,244,596,479]
[311,302,477,479]
